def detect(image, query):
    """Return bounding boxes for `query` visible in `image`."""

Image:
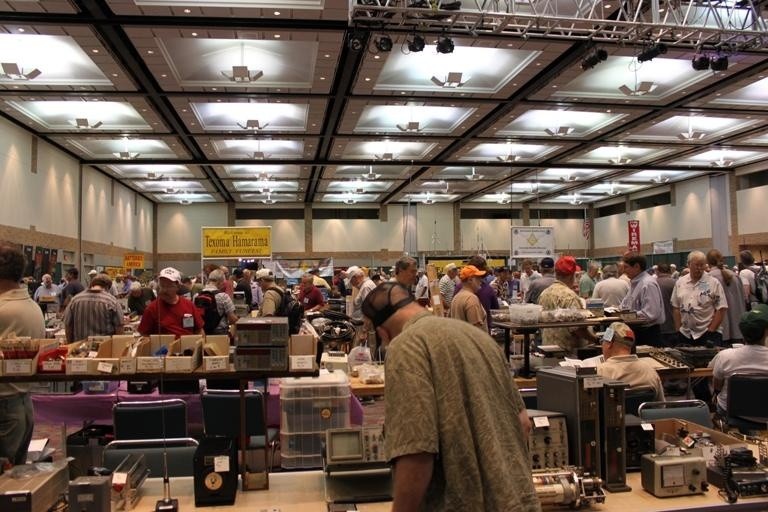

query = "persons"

[1,250,46,468]
[390,256,428,307]
[710,304,767,421]
[439,256,600,346]
[361,281,542,512]
[592,251,767,346]
[32,262,379,342]
[598,321,667,409]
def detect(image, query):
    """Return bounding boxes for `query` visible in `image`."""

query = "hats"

[64,267,78,275]
[739,303,768,337]
[444,255,581,281]
[596,322,635,346]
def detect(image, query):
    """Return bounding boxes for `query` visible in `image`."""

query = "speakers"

[194,437,238,507]
